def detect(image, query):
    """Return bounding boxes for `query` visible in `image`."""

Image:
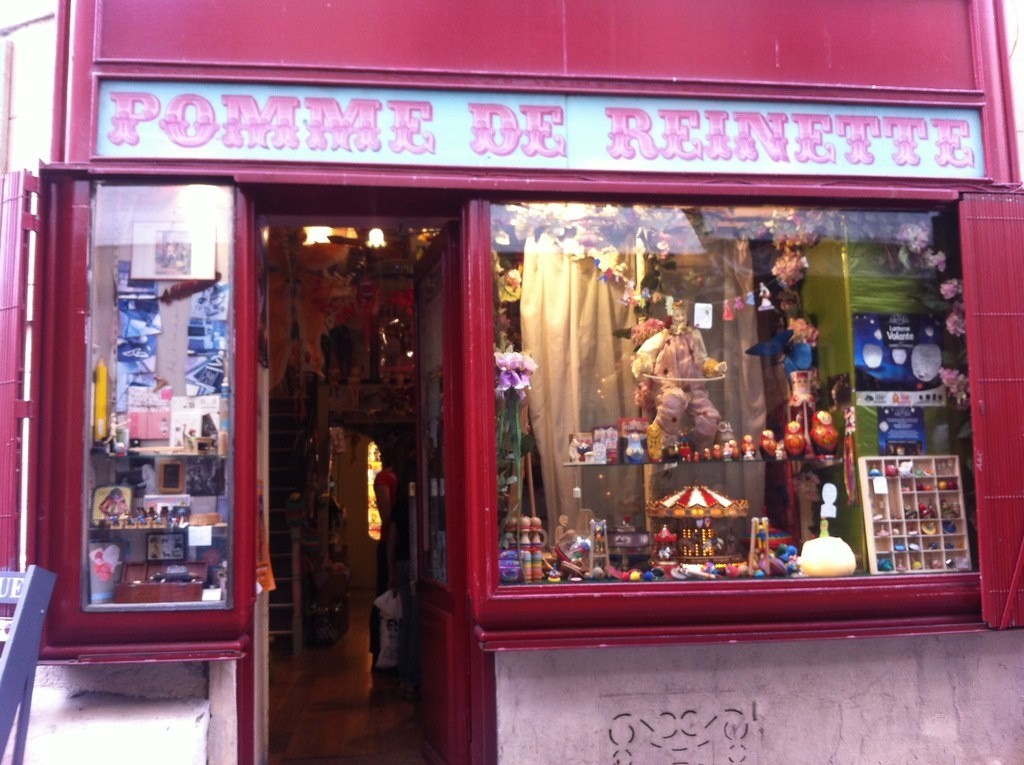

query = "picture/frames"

[128,218,218,280]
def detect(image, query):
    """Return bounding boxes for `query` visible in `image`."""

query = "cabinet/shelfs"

[857,454,972,576]
[80,403,231,610]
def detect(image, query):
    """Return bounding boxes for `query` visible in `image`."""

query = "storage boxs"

[113,560,208,602]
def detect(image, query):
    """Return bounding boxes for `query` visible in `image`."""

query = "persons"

[555,515,575,544]
[369,429,420,702]
[631,300,728,463]
